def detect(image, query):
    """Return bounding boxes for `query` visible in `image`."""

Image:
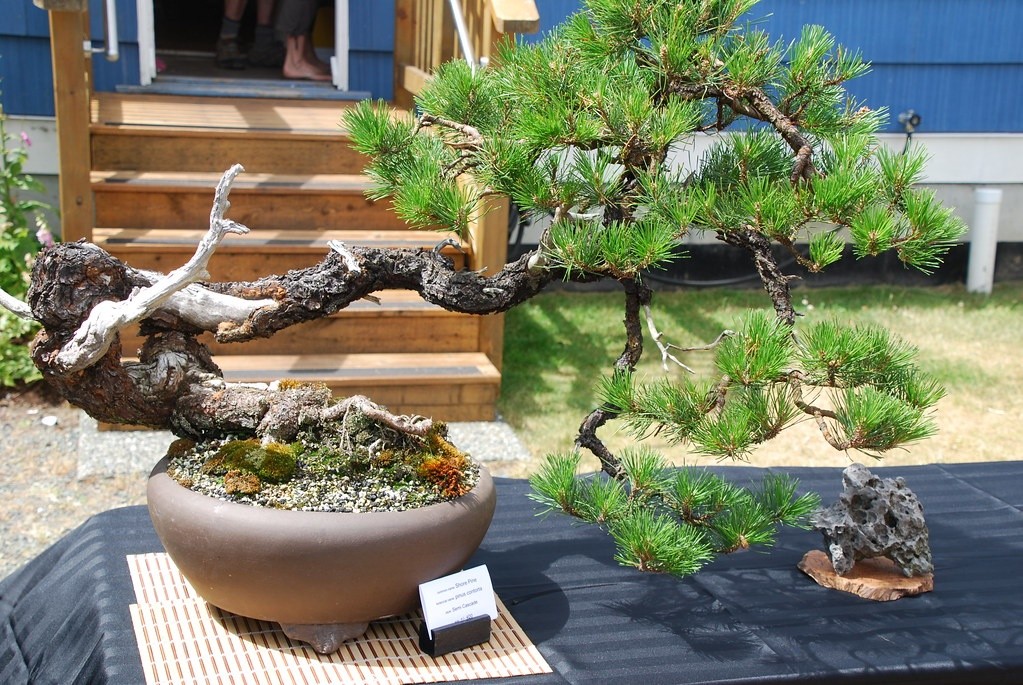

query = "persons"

[276,0,332,81]
[216,0,285,69]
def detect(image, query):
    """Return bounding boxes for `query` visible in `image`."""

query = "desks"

[0,459,1023,685]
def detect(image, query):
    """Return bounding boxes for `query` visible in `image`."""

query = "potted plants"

[0,0,966,656]
